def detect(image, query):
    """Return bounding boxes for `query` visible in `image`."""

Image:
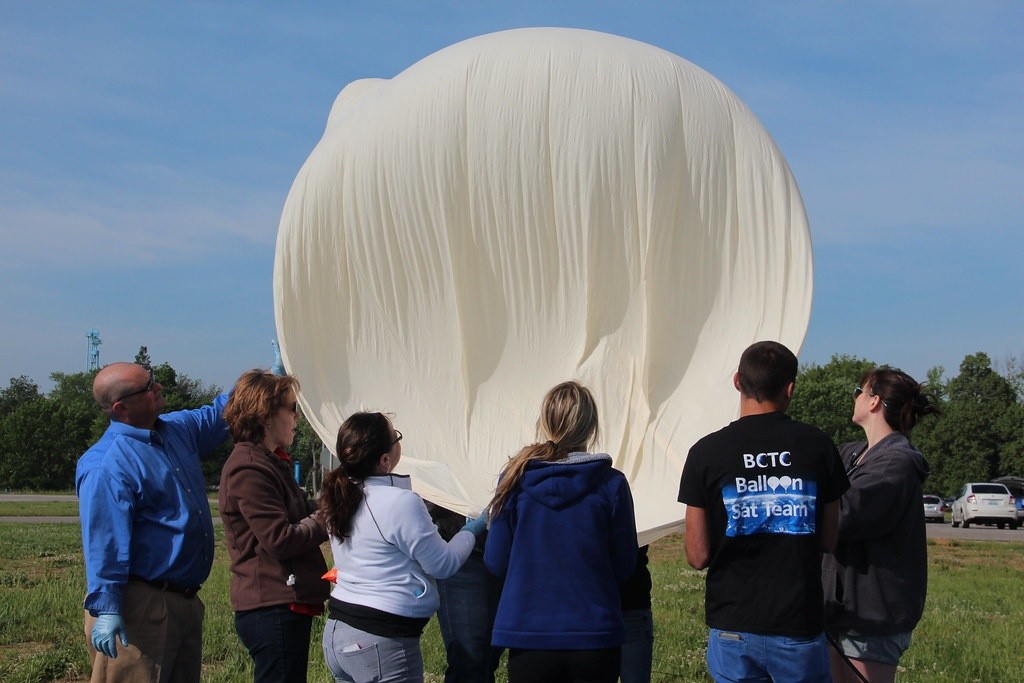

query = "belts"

[133,576,202,599]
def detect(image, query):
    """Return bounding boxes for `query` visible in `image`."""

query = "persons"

[74,338,286,683]
[420,380,653,683]
[218,368,331,683]
[821,364,931,682]
[676,339,852,683]
[323,410,490,683]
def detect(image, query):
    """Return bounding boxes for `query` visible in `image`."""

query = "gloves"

[92,615,129,659]
[461,508,488,537]
[270,340,286,377]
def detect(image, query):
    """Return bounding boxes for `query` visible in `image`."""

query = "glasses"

[279,402,297,413]
[117,369,156,402]
[384,430,402,453]
[852,386,887,408]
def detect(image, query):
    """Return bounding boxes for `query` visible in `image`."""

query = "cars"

[949,482,1019,529]
[942,497,954,508]
[1013,491,1024,527]
[922,494,945,523]
